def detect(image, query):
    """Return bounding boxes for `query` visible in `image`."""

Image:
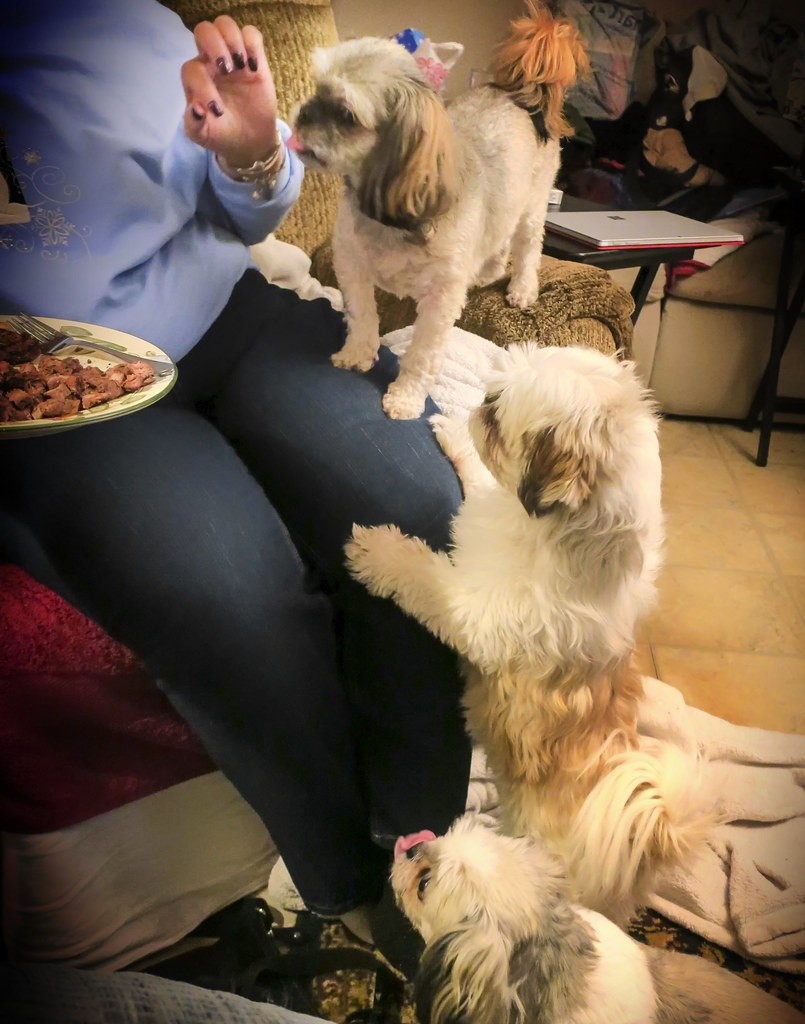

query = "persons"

[0,0,473,982]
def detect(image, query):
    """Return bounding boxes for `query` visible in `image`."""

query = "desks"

[545,233,720,325]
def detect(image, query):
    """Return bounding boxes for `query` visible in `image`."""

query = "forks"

[5,312,183,376]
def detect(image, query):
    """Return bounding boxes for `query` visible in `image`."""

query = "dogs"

[345,339,727,927]
[387,810,720,1024]
[284,0,590,419]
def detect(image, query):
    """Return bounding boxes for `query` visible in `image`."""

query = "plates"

[0,316,178,439]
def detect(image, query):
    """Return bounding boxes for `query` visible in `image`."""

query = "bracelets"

[217,127,285,201]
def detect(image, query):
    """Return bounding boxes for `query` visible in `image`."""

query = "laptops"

[544,209,745,250]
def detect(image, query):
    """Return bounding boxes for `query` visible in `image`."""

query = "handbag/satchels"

[638,42,746,188]
[549,0,658,120]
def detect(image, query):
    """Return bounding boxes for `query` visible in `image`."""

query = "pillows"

[0,962,337,1024]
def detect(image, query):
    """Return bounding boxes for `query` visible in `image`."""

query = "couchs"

[0,0,804,1024]
